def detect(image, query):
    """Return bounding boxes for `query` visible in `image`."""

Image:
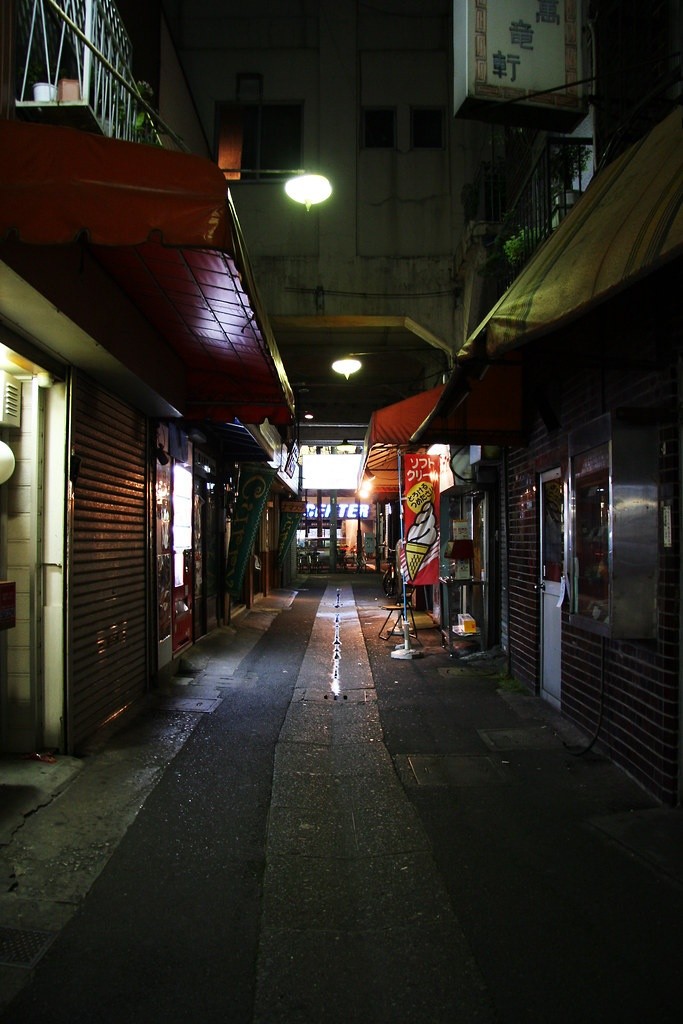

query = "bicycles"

[297,547,304,574]
[303,546,323,576]
[351,546,366,573]
[378,544,396,597]
[337,545,348,571]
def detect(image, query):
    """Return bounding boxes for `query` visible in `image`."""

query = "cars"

[297,536,353,565]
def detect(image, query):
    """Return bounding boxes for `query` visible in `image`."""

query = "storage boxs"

[458,613,476,634]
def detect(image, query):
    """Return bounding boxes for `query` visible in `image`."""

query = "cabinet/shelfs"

[439,577,487,660]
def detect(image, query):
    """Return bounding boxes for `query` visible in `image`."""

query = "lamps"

[336,440,356,451]
[331,350,381,380]
[222,166,334,212]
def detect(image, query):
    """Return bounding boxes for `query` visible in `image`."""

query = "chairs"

[378,585,418,641]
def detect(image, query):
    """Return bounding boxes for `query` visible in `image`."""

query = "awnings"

[365,357,525,473]
[463,105,683,357]
[2,113,294,427]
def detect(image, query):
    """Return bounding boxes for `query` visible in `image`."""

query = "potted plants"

[20,65,58,102]
[461,218,497,276]
[58,63,79,101]
